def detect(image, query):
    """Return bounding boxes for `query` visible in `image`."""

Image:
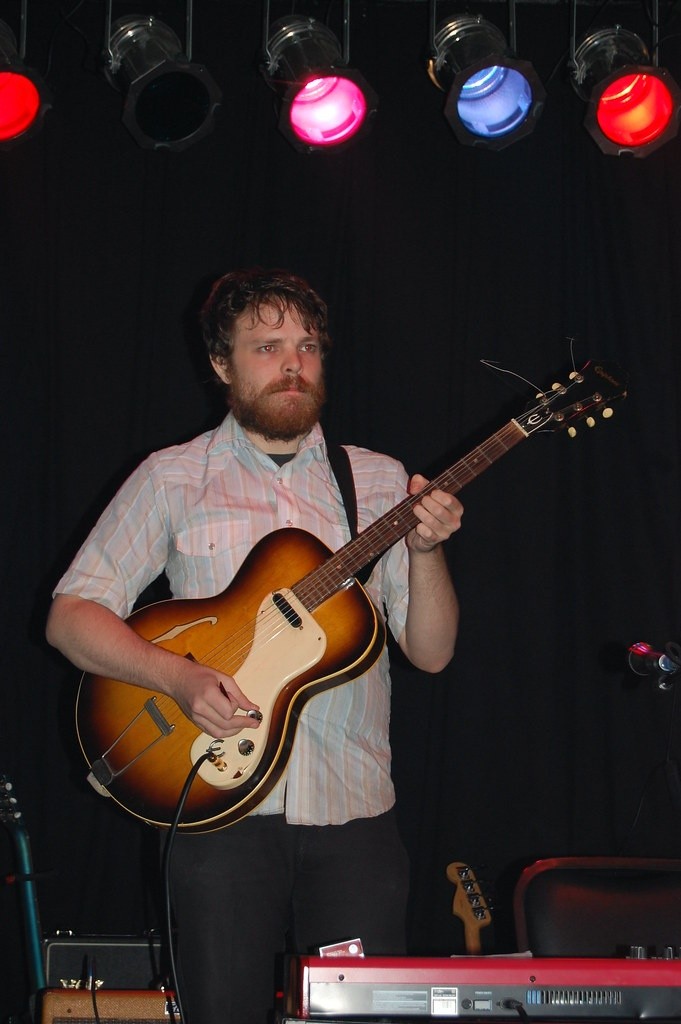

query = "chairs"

[513,858,681,959]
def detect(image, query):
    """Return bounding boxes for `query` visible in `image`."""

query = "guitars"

[72,360,629,835]
[446,862,493,957]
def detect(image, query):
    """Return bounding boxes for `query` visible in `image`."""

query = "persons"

[44,265,465,1024]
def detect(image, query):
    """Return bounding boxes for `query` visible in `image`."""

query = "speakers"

[34,931,184,1024]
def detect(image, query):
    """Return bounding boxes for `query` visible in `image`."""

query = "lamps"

[565,0,681,159]
[428,0,550,153]
[103,0,222,150]
[258,0,377,156]
[0,0,55,153]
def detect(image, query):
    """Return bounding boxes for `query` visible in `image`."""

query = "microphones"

[628,643,679,675]
